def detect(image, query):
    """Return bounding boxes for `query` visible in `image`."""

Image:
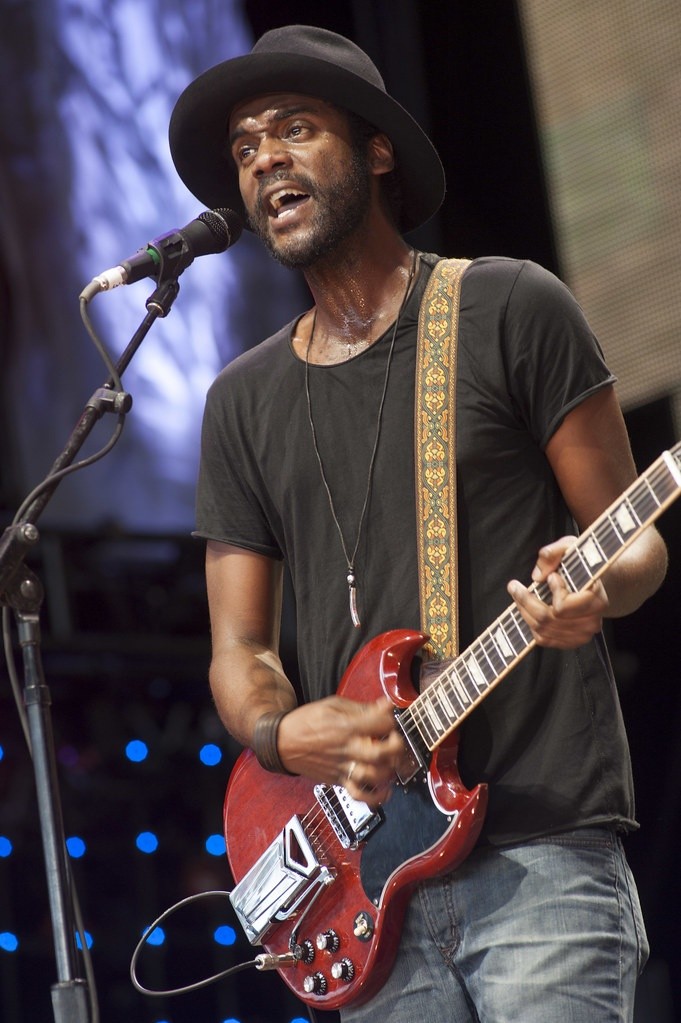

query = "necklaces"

[305,246,418,627]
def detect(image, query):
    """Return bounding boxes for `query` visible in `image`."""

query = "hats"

[168,23,449,233]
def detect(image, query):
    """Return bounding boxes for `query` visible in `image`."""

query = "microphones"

[80,208,243,301]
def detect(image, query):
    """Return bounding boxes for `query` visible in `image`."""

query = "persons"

[168,25,669,1023]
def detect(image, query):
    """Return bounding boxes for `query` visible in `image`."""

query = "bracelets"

[254,710,301,777]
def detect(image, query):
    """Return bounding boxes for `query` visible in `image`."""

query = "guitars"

[220,438,680,1009]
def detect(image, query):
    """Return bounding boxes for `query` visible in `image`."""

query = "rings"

[347,761,356,780]
